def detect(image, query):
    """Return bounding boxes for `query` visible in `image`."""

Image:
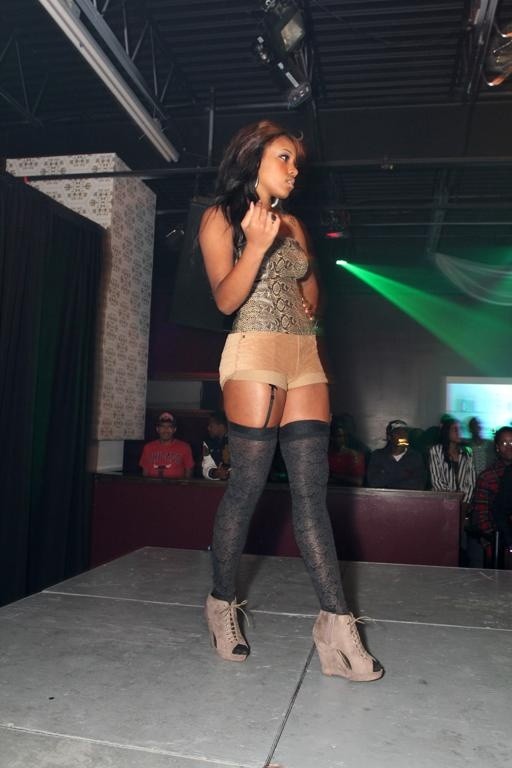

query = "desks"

[88,467,465,567]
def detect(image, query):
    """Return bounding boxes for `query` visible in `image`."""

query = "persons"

[202,411,287,480]
[138,411,196,480]
[329,409,512,570]
[198,119,387,683]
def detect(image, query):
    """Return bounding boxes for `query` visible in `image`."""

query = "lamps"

[263,1,308,57]
[267,55,313,111]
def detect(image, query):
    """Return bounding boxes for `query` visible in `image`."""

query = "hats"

[156,412,175,423]
[386,417,409,432]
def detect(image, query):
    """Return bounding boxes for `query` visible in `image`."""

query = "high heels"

[204,592,250,663]
[311,609,384,681]
